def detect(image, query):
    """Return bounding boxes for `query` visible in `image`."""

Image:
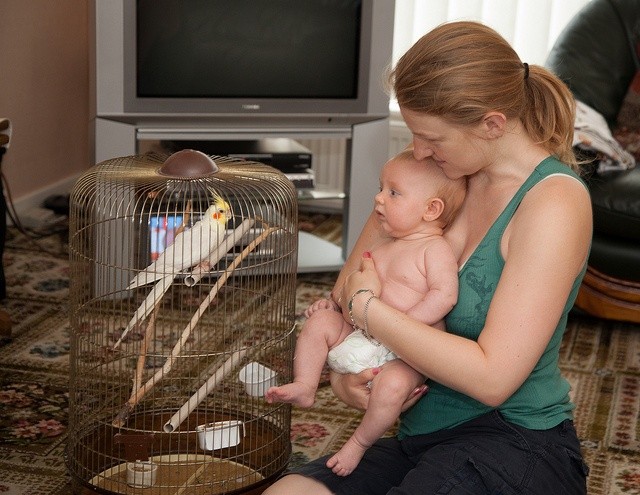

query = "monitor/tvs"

[94,0,395,120]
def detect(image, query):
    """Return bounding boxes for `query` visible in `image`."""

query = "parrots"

[110,187,232,351]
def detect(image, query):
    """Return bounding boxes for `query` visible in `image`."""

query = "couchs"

[529,0,640,324]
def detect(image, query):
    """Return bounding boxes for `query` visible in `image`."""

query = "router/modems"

[189,225,274,256]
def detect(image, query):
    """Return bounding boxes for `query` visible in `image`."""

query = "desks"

[92,114,390,302]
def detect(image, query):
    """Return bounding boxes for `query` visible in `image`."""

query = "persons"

[258,21,593,494]
[265,149,469,479]
